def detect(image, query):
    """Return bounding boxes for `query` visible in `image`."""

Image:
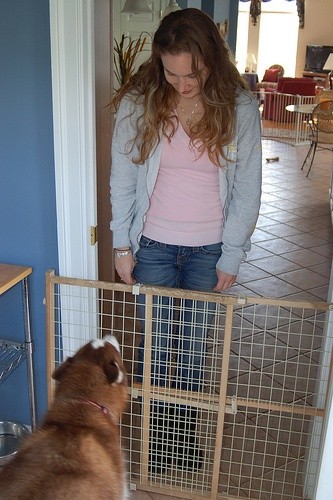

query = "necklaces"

[179,100,199,125]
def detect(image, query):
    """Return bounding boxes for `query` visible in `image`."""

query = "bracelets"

[114,247,131,257]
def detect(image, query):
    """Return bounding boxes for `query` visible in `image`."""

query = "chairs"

[301,89,333,178]
[261,64,285,82]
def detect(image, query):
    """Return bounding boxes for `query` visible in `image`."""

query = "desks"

[257,82,278,90]
[285,103,333,141]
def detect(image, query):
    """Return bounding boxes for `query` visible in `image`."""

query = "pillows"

[263,69,279,81]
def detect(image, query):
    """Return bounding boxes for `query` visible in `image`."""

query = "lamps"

[163,0,183,18]
[120,0,153,14]
[322,53,333,90]
[245,52,257,73]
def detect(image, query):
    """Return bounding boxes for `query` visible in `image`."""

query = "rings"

[121,278,125,280]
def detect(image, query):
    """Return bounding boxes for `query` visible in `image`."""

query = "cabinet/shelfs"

[0,264,38,470]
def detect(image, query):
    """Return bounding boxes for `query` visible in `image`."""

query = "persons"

[108,7,263,473]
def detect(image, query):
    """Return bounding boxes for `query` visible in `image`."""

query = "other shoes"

[150,434,172,472]
[173,431,202,468]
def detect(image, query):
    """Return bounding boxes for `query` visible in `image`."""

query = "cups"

[252,63,256,73]
[315,86,324,95]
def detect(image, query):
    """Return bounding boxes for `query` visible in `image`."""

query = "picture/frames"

[127,1,154,23]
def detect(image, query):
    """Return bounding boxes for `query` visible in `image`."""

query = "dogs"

[0,334,130,500]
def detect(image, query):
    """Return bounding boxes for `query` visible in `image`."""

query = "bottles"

[245,67,249,73]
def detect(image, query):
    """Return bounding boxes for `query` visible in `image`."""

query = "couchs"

[242,74,260,108]
[262,76,319,122]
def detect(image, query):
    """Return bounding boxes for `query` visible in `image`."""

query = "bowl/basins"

[0,420,31,466]
